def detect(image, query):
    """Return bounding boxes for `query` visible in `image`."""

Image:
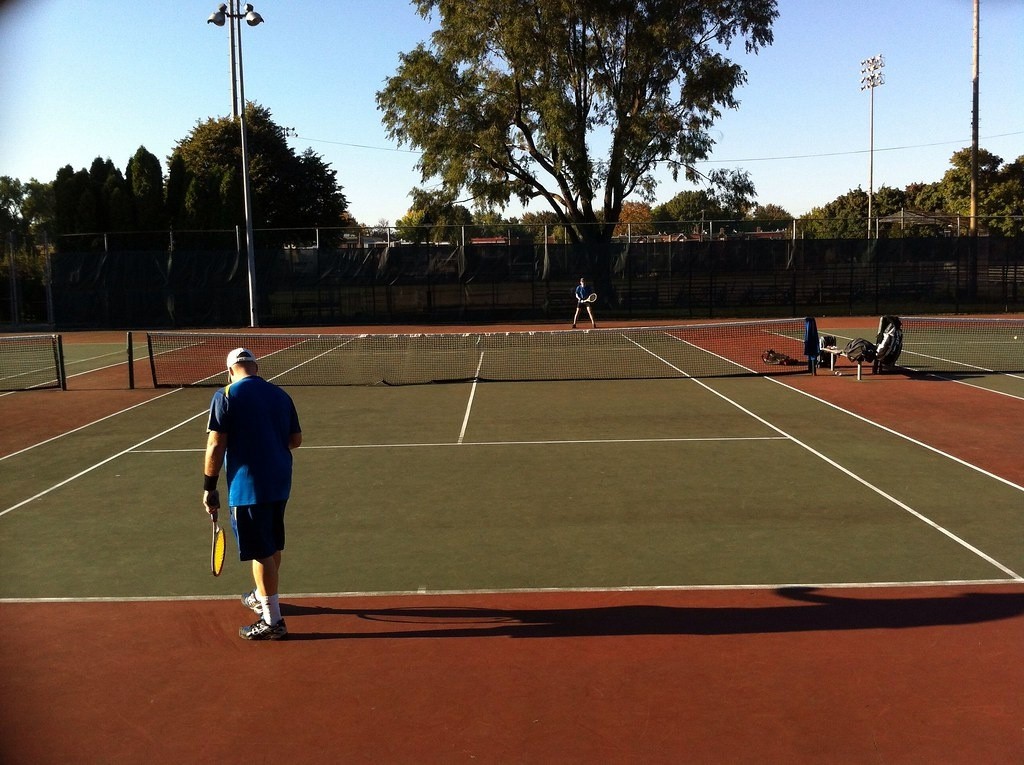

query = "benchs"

[822,347,864,381]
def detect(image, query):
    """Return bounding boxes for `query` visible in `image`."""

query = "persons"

[571,277,597,329]
[203,348,301,639]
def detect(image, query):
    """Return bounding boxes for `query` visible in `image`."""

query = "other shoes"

[572,324,576,328]
[591,324,596,328]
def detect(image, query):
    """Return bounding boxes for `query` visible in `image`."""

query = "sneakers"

[241,589,263,617]
[239,618,289,640]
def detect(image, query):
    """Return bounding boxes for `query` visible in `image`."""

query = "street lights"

[207,0,266,327]
[861,53,886,279]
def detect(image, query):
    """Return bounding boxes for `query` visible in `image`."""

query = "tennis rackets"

[209,490,227,578]
[583,293,597,302]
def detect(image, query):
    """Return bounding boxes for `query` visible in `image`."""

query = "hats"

[227,347,257,384]
